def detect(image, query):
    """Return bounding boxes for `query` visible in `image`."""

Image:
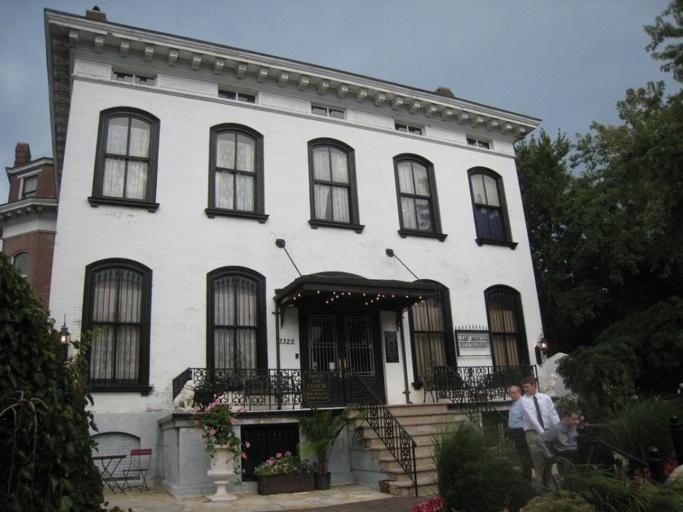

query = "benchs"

[422,373,464,402]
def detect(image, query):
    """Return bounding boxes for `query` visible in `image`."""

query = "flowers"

[253,449,313,476]
[192,399,250,486]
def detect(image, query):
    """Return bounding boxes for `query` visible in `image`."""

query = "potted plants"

[290,397,370,490]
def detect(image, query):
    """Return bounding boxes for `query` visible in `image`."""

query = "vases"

[204,444,241,500]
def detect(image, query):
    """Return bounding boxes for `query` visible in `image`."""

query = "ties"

[533,396,544,430]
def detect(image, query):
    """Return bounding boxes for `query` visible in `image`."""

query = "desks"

[90,454,127,495]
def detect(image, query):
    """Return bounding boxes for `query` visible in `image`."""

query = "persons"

[508,376,581,485]
[664,463,683,493]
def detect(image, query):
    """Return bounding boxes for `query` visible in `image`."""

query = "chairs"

[119,448,152,493]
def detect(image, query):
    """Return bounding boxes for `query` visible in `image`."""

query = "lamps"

[57,312,71,361]
[535,331,549,366]
